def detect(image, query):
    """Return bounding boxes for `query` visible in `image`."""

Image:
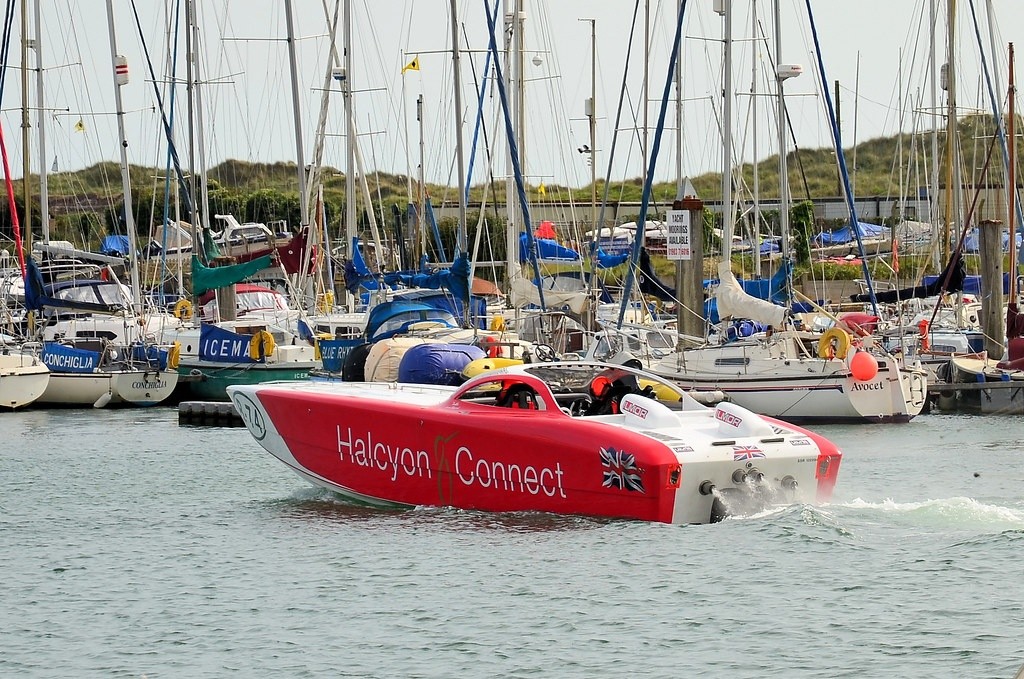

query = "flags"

[403,58,418,73]
[75,120,85,132]
[539,183,546,196]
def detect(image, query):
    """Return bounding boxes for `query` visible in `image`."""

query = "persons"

[584,350,656,414]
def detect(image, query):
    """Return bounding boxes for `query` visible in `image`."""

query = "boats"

[223,362,843,524]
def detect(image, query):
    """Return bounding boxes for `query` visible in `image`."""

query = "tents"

[820,218,952,252]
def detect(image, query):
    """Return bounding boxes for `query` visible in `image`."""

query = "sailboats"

[0,0,1024,415]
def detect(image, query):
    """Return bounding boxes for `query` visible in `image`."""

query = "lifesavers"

[320,293,333,314]
[819,327,850,359]
[490,315,506,331]
[644,297,663,316]
[168,340,181,369]
[480,336,504,359]
[174,300,194,318]
[250,331,275,359]
